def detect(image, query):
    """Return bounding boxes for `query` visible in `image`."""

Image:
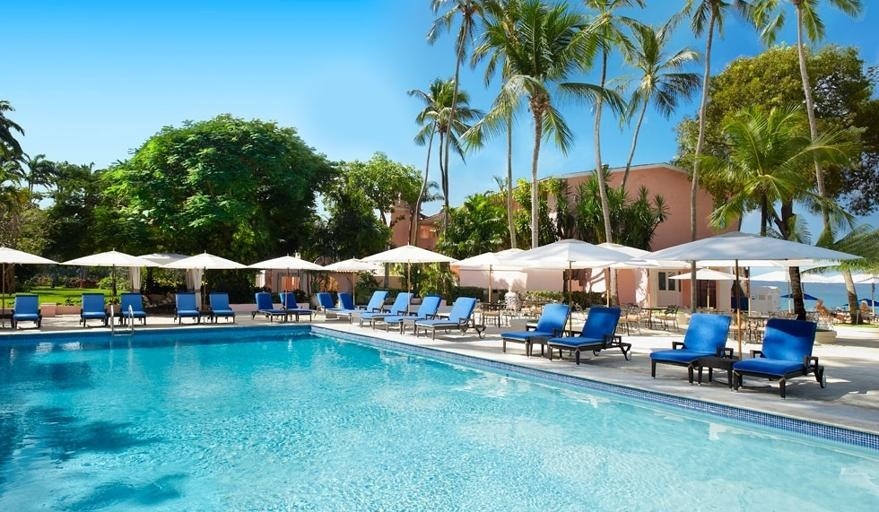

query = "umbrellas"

[248,251,328,323]
[324,253,378,309]
[163,248,248,323]
[360,240,459,315]
[0,243,60,328]
[59,245,159,304]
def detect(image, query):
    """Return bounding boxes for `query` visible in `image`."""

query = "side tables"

[0,309,13,329]
